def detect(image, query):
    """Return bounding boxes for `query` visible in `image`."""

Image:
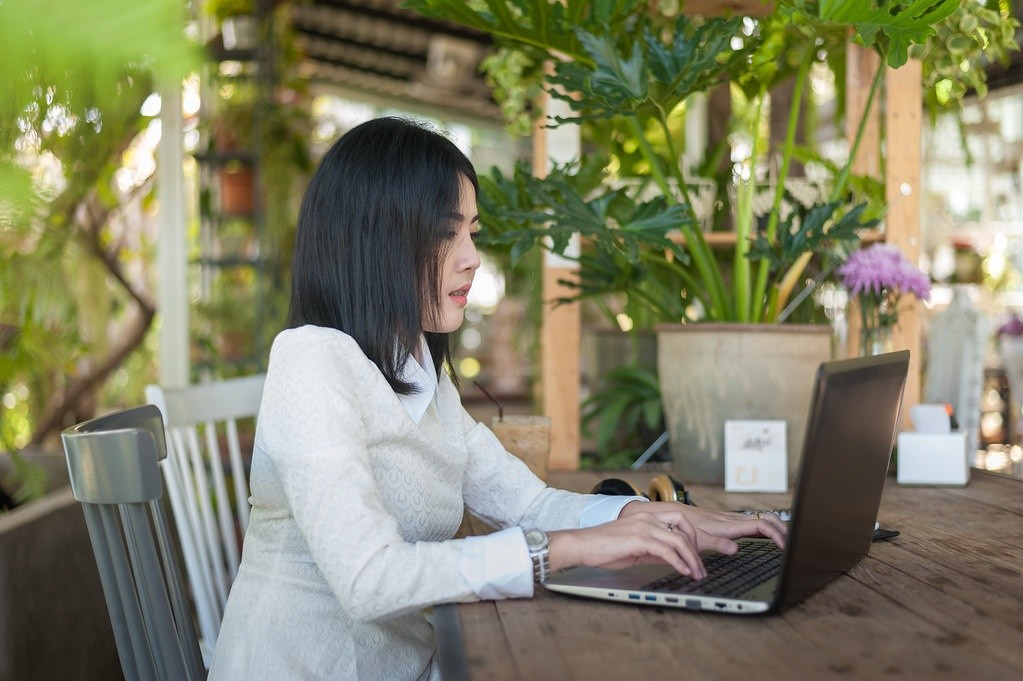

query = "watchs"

[524,527,553,581]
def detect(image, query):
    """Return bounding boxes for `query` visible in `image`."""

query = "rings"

[667,523,672,530]
[756,511,762,518]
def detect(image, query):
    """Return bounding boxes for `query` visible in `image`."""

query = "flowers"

[836,242,931,357]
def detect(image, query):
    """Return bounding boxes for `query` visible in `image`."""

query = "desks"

[430,461,1023,681]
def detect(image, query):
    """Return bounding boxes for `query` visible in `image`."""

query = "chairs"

[144,372,267,656]
[63,404,206,681]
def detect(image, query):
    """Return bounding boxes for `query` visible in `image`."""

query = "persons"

[207,119,788,681]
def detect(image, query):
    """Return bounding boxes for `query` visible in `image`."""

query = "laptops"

[543,349,910,616]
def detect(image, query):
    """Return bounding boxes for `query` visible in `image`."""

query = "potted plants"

[192,42,315,215]
[395,0,1021,487]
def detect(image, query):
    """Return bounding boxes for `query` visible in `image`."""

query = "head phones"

[589,475,693,506]
